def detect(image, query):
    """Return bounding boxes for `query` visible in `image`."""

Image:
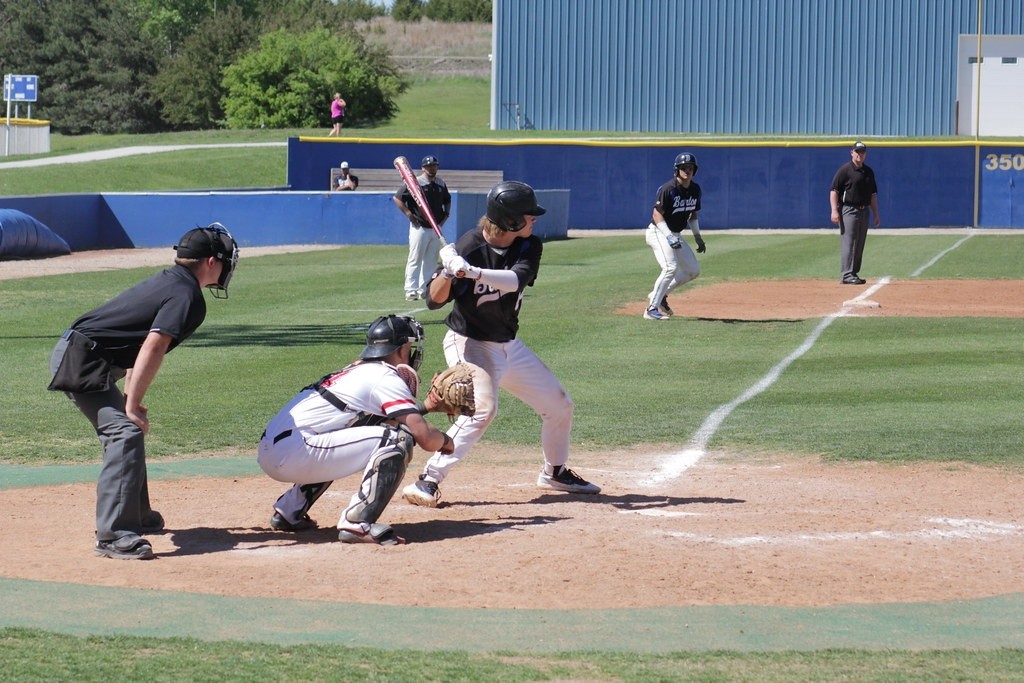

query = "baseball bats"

[393,155,465,278]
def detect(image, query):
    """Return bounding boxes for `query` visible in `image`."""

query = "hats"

[341,162,349,170]
[852,142,866,151]
[176,228,234,259]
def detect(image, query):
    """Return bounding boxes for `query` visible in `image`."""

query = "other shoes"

[406,290,419,300]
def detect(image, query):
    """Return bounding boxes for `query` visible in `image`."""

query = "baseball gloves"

[426,360,487,430]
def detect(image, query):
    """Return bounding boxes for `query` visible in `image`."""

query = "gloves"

[667,234,682,249]
[694,234,706,253]
[438,243,482,280]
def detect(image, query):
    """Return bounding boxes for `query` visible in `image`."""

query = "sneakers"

[270,512,318,531]
[537,465,601,494]
[339,528,399,546]
[644,309,671,320]
[139,511,164,533]
[404,474,441,508]
[95,533,152,561]
[649,290,673,315]
[844,276,866,284]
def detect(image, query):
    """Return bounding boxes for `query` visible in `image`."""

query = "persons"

[331,162,358,191]
[644,153,706,320]
[47,222,240,561]
[258,312,475,546]
[830,142,880,285]
[393,155,450,301]
[330,93,347,137]
[403,180,602,507]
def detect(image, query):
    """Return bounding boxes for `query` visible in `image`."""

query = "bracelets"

[439,433,449,452]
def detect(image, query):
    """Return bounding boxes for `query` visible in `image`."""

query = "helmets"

[674,152,698,176]
[360,314,413,359]
[486,180,547,233]
[422,156,439,166]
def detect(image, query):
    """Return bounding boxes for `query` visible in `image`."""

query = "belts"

[262,427,293,444]
